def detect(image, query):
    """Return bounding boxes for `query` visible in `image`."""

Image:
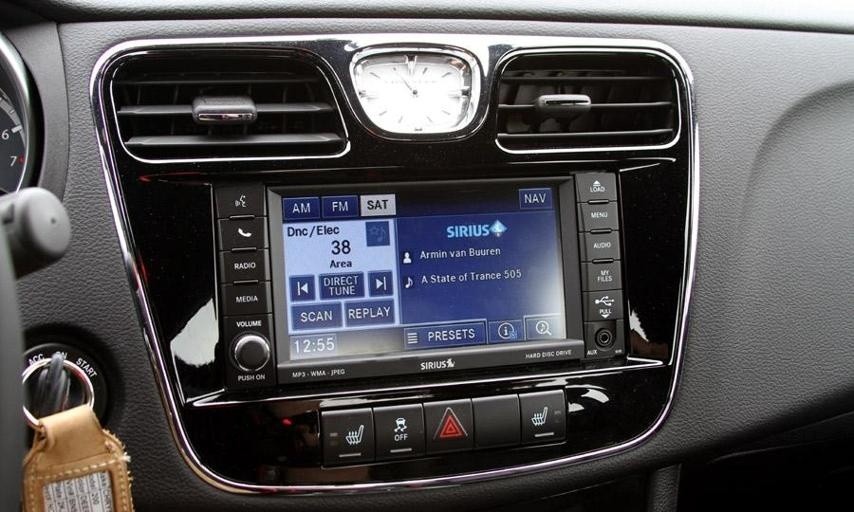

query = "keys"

[31,351,70,419]
